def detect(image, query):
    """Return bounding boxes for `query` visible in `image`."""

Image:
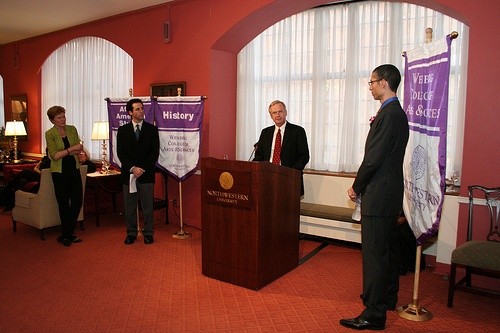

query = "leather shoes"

[144,235,153,244]
[125,235,136,244]
[340,315,385,330]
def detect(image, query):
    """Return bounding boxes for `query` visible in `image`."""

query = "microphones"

[248,144,257,161]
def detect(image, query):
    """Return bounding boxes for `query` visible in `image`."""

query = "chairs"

[446,185,500,308]
[11,165,88,241]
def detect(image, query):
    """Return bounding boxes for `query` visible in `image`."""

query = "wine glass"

[0,150,10,163]
[451,169,460,192]
[78,135,85,155]
[105,161,110,171]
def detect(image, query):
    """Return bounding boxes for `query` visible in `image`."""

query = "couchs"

[0,163,38,212]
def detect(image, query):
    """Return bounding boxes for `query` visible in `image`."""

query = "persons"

[34,145,96,191]
[339,64,410,331]
[252,100,310,196]
[116,99,160,244]
[45,106,83,247]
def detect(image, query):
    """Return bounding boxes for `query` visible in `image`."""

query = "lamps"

[4,120,27,163]
[90,121,111,174]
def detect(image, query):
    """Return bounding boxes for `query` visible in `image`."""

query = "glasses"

[79,153,86,156]
[368,78,387,86]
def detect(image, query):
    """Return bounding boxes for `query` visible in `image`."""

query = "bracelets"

[66,149,69,154]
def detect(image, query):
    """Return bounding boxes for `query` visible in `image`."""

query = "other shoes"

[398,264,407,274]
[72,233,81,242]
[58,238,71,246]
[4,206,11,211]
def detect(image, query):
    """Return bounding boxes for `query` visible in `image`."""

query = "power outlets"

[169,199,179,206]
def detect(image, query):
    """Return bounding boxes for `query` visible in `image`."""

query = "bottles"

[352,193,361,221]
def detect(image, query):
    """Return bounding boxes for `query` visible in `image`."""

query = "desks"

[0,158,122,229]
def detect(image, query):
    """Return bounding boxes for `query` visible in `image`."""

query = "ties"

[136,124,139,141]
[272,129,281,164]
